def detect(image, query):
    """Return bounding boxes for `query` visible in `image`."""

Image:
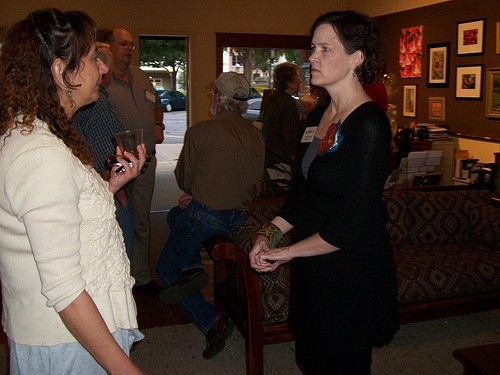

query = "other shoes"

[135,280,164,292]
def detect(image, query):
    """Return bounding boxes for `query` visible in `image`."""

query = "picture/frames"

[454,64,484,100]
[402,84,418,118]
[485,68,500,118]
[428,97,446,123]
[425,42,451,88]
[456,17,486,57]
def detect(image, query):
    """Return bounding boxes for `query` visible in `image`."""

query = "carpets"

[0,308,500,375]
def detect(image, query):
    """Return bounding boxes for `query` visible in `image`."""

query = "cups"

[113,128,143,163]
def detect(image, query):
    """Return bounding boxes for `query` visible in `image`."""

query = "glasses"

[111,39,137,51]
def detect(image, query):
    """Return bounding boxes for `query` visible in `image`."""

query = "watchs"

[156,122,165,130]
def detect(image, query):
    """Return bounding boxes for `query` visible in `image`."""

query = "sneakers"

[203,317,234,359]
[159,266,209,305]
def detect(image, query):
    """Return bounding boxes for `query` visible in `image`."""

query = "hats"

[214,71,250,101]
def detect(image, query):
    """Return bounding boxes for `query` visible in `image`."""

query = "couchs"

[199,179,500,375]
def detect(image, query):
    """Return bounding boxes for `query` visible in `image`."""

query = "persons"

[260,62,303,194]
[0,9,146,375]
[100,28,164,297]
[154,70,265,358]
[72,42,134,263]
[248,10,399,375]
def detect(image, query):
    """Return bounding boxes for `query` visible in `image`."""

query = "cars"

[157,89,185,112]
[245,97,262,118]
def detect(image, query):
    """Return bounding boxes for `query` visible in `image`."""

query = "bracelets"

[253,222,283,248]
